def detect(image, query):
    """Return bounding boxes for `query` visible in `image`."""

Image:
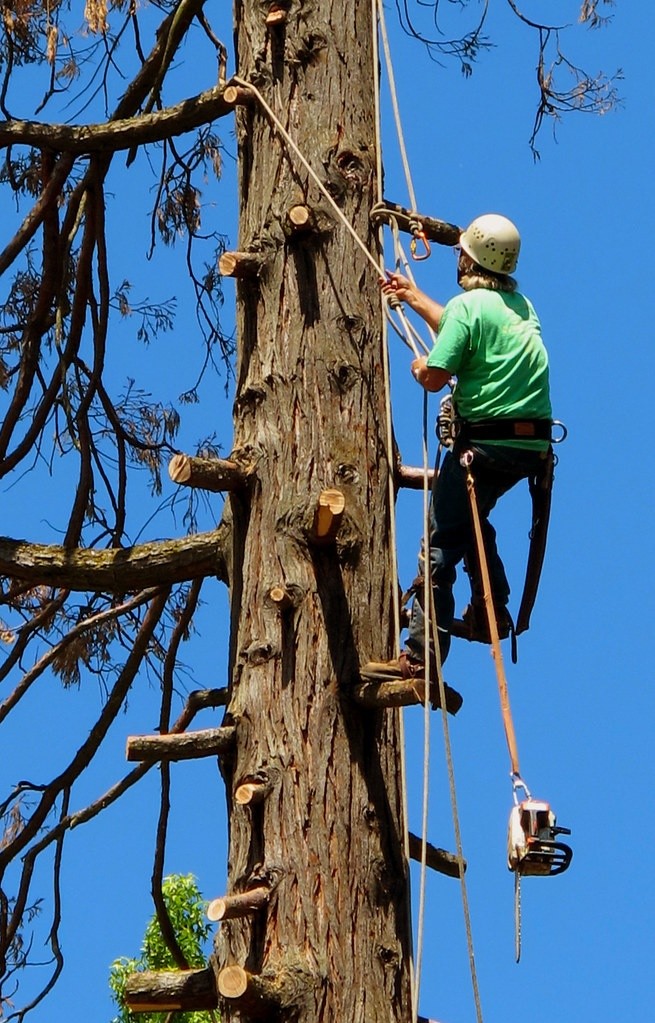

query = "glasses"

[453,245,463,257]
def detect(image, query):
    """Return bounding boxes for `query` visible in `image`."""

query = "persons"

[357,214,554,681]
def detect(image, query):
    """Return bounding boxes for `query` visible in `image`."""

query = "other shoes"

[359,654,435,684]
[464,599,510,640]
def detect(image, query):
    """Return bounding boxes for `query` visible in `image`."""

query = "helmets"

[458,214,521,274]
